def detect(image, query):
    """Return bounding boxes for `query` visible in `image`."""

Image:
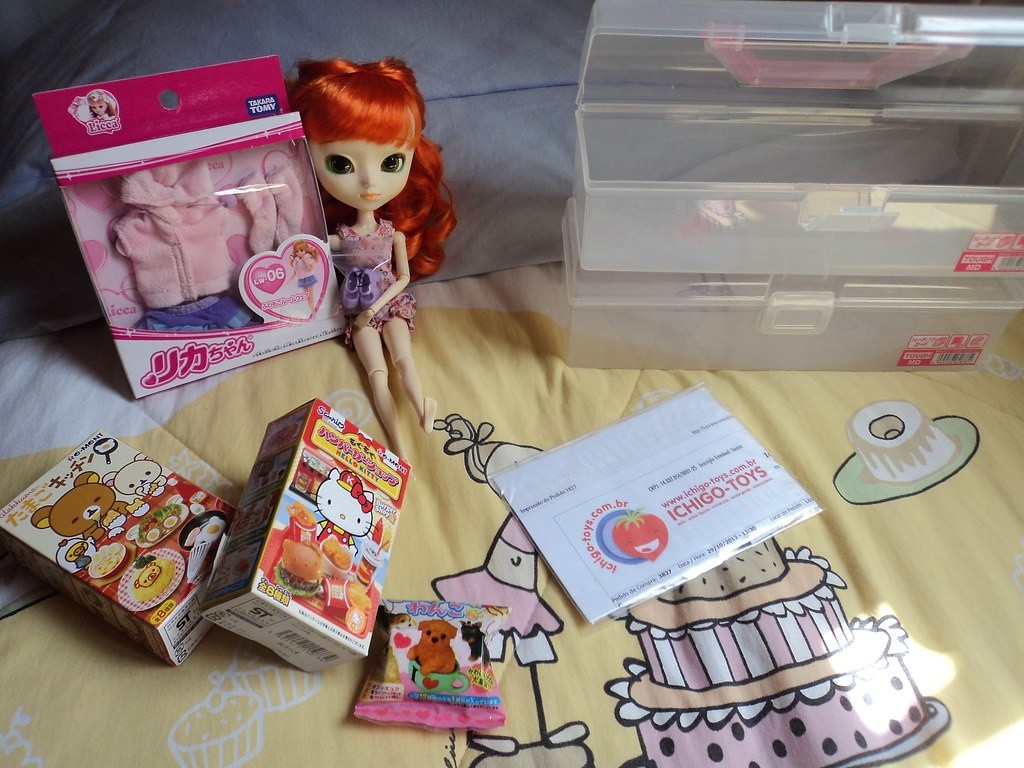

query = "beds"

[0,259,1024,768]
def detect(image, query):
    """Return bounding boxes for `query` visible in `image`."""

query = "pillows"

[0,0,963,343]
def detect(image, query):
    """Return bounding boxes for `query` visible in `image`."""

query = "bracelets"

[367,308,376,314]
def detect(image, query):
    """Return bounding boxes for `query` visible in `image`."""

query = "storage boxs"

[571,0,1024,280]
[32,53,346,399]
[199,396,412,675]
[560,195,1024,373]
[0,428,236,667]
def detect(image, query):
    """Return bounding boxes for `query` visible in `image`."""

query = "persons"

[286,54,458,474]
